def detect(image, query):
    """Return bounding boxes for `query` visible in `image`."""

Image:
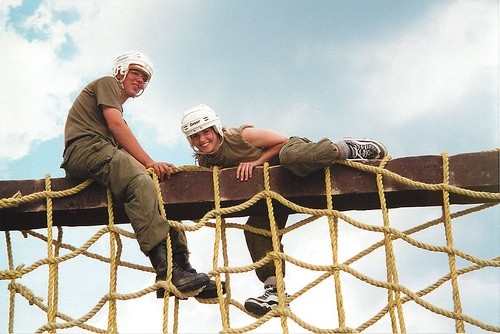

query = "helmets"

[111,51,153,98]
[180,104,224,155]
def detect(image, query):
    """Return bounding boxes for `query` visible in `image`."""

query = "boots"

[167,227,228,298]
[148,239,209,297]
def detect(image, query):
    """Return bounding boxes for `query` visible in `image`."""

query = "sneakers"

[341,137,387,159]
[246,289,291,312]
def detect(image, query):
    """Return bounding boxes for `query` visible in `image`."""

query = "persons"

[60,50,229,300]
[182,103,387,317]
